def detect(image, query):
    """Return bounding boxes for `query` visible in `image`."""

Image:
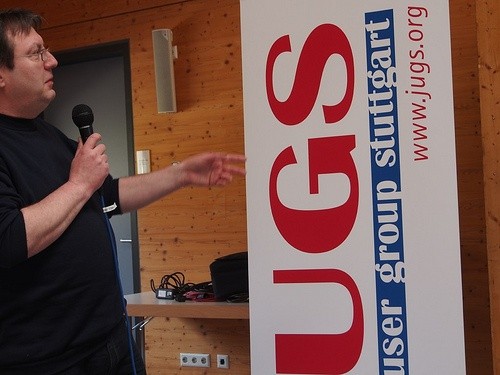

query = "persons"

[0,8,248,375]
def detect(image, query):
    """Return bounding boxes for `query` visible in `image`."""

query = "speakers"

[152,28,177,113]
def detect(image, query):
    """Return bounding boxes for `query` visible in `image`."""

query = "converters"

[156,288,175,300]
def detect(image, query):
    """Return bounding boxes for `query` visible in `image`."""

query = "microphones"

[71,105,104,196]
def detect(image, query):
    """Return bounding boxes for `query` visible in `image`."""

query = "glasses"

[12,45,52,63]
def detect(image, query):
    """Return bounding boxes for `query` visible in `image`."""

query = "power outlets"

[180,353,210,367]
[217,355,229,368]
